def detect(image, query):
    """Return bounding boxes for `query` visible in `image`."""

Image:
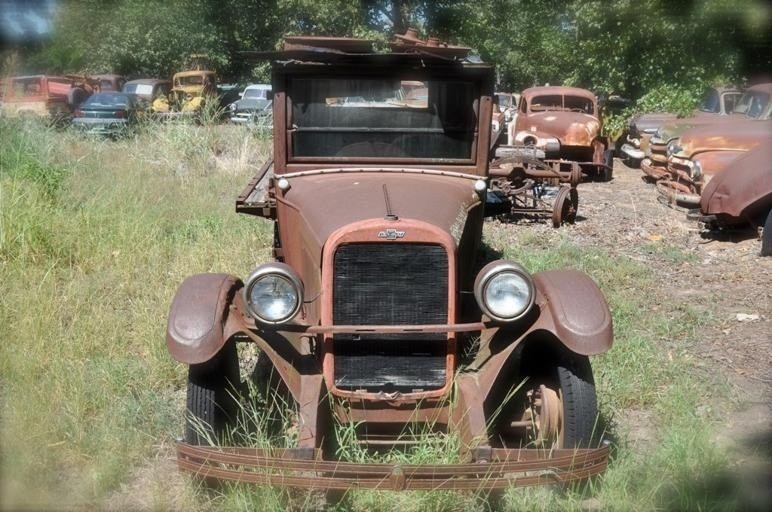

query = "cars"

[1,64,218,138]
[616,80,772,258]
